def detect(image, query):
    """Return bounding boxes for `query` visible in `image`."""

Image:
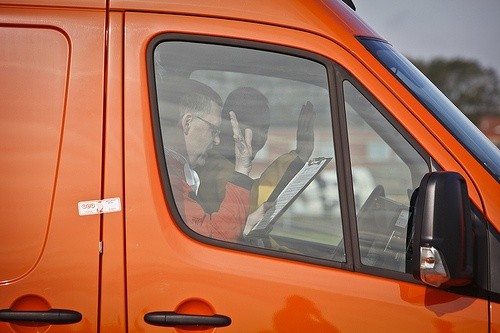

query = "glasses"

[190,114,222,137]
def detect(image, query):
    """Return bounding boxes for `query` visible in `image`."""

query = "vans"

[0,0,500,332]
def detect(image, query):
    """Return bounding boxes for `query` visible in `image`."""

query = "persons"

[195,86,316,255]
[157,77,279,241]
